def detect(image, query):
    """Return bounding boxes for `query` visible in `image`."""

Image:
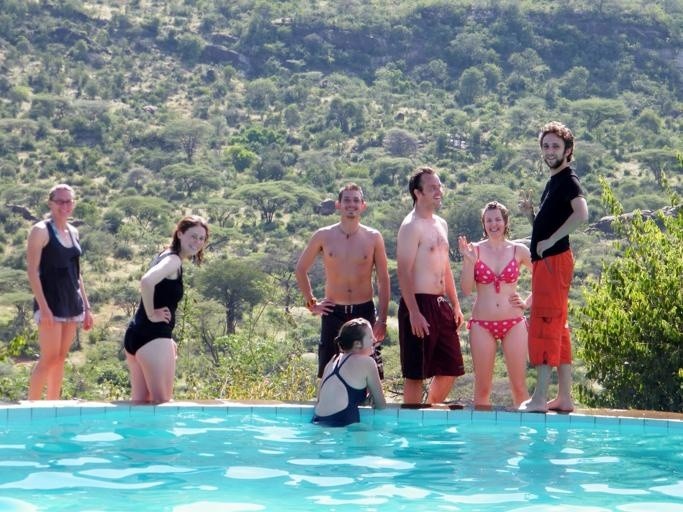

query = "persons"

[124,215,212,407]
[26,182,95,402]
[516,121,590,413]
[458,200,533,407]
[397,165,465,402]
[296,183,391,408]
[310,318,388,429]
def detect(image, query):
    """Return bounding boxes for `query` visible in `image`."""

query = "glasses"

[50,199,74,206]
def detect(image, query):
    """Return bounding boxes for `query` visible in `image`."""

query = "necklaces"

[338,226,359,240]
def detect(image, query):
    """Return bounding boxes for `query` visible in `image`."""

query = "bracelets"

[377,320,388,328]
[305,297,318,308]
[85,304,92,311]
